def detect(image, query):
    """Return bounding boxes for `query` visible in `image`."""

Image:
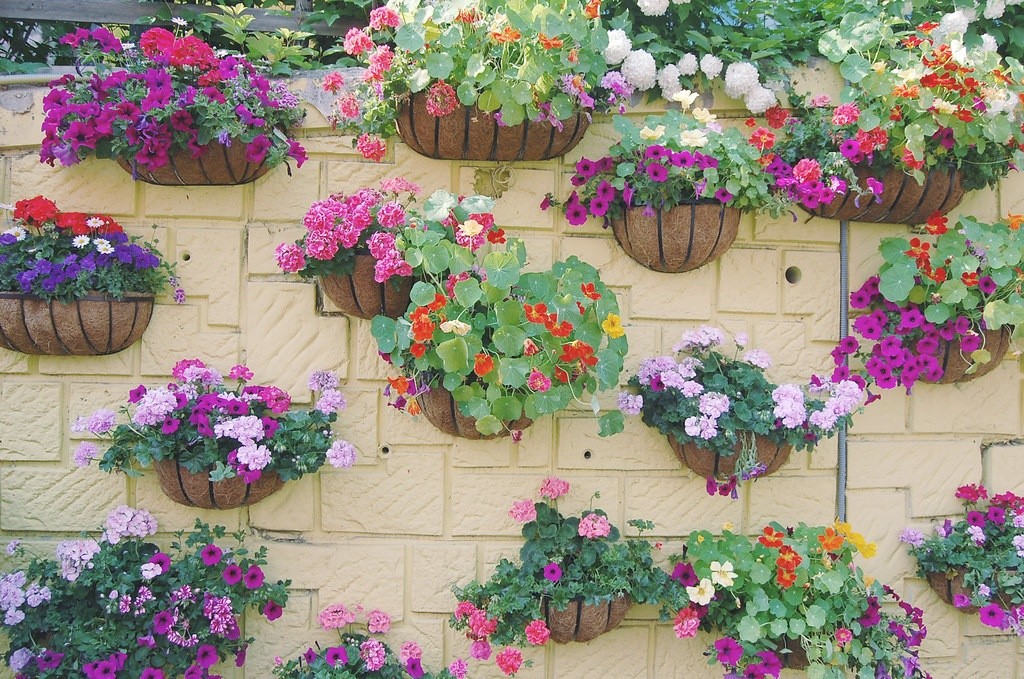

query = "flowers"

[539,14,1024,227]
[671,516,933,679]
[618,323,867,502]
[448,475,700,676]
[318,0,631,161]
[274,177,626,444]
[38,26,307,177]
[0,195,186,306]
[271,603,492,679]
[0,506,292,679]
[830,211,1024,405]
[899,482,1024,642]
[70,358,356,483]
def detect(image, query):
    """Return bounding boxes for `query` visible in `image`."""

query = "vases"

[397,86,593,161]
[114,140,274,186]
[796,162,965,225]
[666,429,793,481]
[610,208,741,273]
[311,255,420,319]
[906,324,1012,386]
[924,567,1023,614]
[764,633,810,670]
[411,370,541,443]
[153,458,286,510]
[1,290,156,357]
[534,595,632,644]
